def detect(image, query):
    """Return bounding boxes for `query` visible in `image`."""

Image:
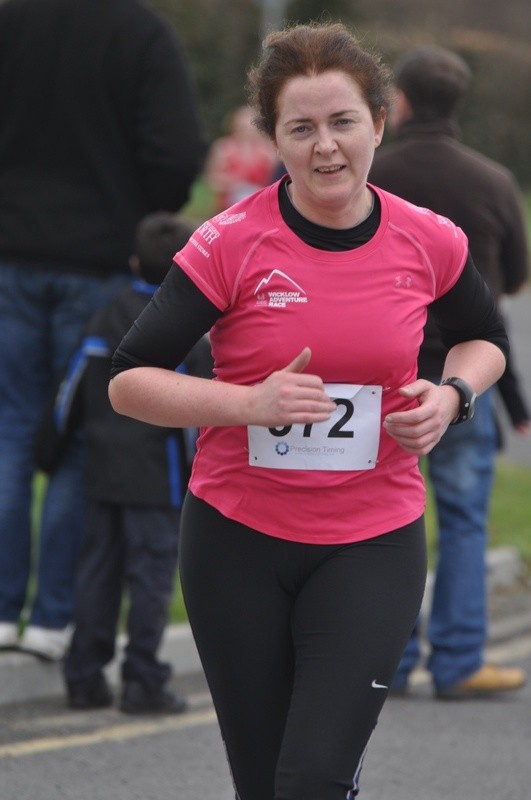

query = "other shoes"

[435,663,527,701]
[17,622,78,661]
[0,621,20,648]
[70,681,114,710]
[120,679,184,716]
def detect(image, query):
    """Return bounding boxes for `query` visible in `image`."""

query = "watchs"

[440,376,478,427]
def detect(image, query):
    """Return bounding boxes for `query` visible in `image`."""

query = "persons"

[106,21,510,800]
[38,211,214,717]
[365,45,531,703]
[204,101,277,216]
[0,0,206,665]
